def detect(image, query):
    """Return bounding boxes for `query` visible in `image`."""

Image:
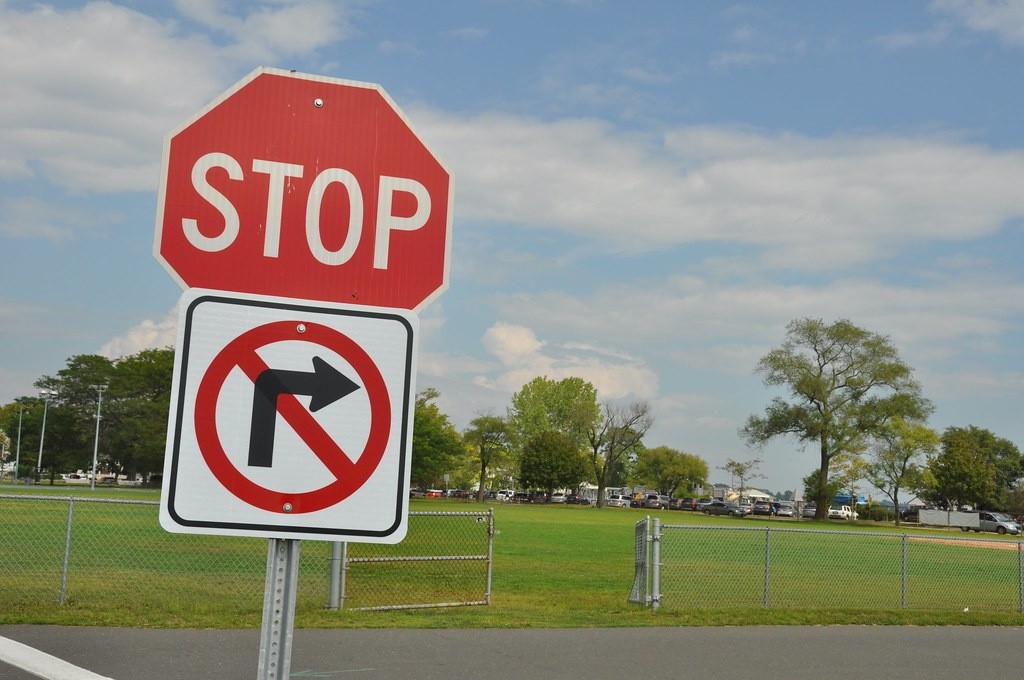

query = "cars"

[777,506,799,517]
[961,510,1022,535]
[410,478,779,518]
[802,503,816,517]
[827,503,860,521]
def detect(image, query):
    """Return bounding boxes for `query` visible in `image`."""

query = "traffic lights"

[34,390,59,485]
[87,384,109,489]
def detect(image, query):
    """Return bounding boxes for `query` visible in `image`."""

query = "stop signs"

[152,66,452,315]
